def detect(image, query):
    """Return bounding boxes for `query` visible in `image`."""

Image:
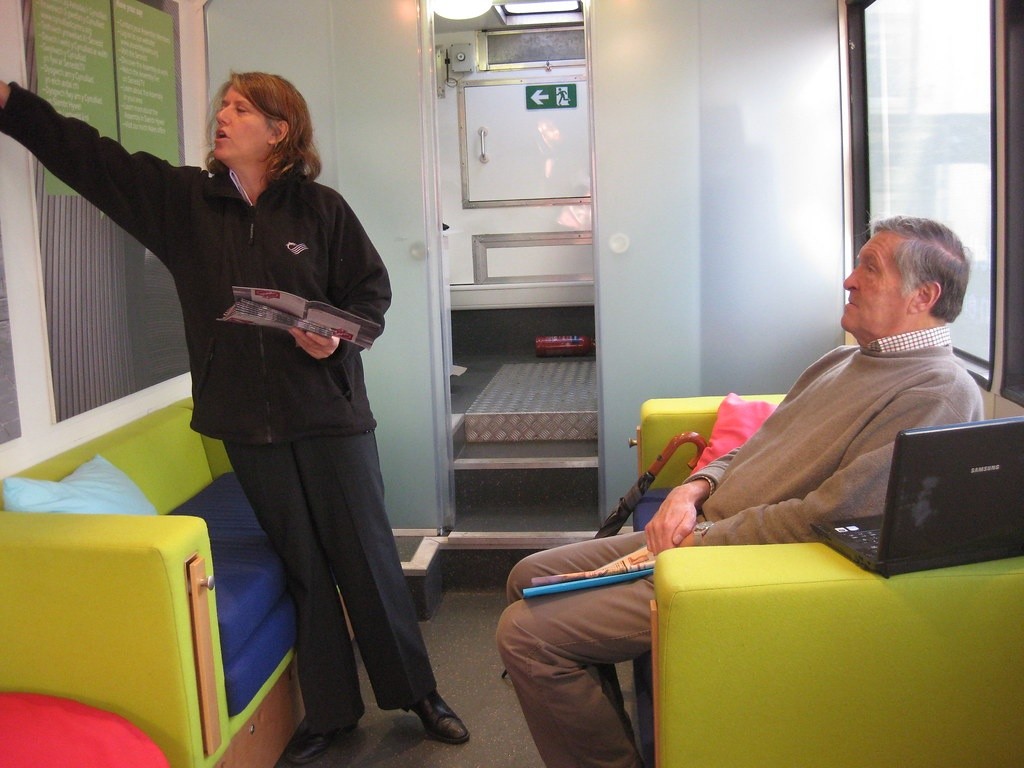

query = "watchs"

[691,520,714,546]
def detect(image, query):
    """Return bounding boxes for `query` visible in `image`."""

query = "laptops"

[809,416,1024,579]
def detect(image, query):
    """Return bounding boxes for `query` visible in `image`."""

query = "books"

[233,284,382,352]
[214,299,336,341]
[522,541,658,600]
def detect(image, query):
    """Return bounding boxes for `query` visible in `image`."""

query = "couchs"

[625,393,1024,765]
[0,394,310,768]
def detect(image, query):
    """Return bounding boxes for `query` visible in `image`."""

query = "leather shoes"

[402,692,470,743]
[287,717,359,762]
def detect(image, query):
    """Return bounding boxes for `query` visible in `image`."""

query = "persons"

[0,70,471,767]
[492,218,986,768]
